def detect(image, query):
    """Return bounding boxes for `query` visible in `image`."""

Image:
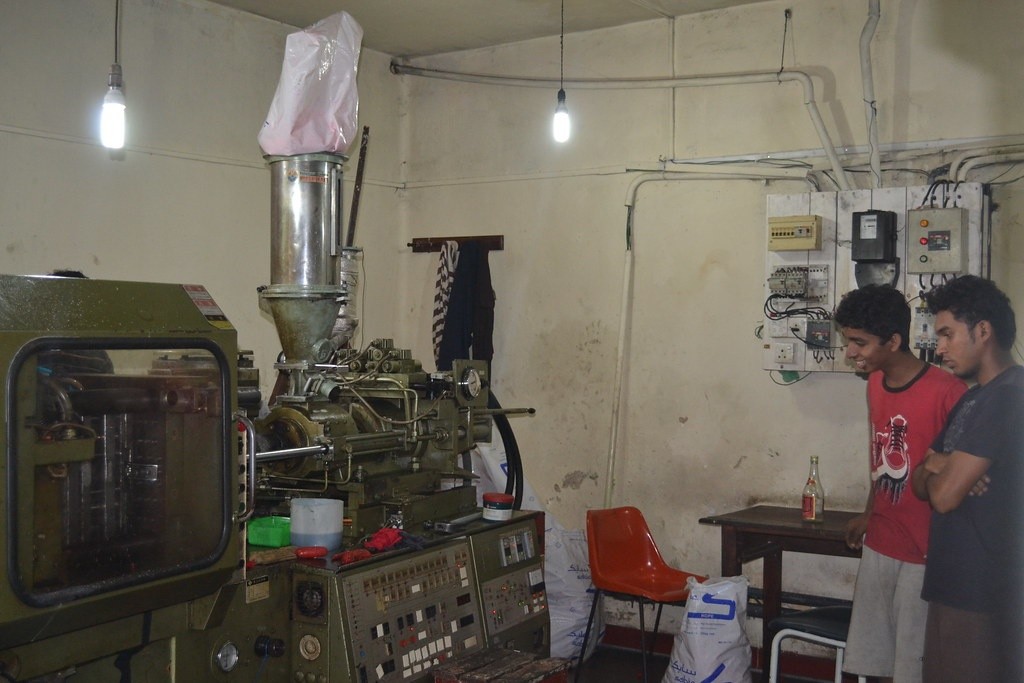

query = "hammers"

[423,509,483,534]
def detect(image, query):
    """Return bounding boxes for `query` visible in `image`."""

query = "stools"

[768,604,853,683]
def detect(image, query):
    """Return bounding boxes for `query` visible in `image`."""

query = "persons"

[910,274,1024,683]
[833,284,969,683]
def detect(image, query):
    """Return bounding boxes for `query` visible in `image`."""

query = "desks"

[698,504,867,683]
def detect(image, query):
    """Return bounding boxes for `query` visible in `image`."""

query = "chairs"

[574,506,707,682]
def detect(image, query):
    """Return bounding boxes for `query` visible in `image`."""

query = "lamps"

[550,0,572,143]
[98,1,126,152]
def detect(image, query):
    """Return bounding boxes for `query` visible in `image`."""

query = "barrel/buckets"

[289,498,344,559]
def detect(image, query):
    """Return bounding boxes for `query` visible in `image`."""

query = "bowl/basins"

[247,516,291,546]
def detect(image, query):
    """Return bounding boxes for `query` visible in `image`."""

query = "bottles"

[802,456,824,522]
